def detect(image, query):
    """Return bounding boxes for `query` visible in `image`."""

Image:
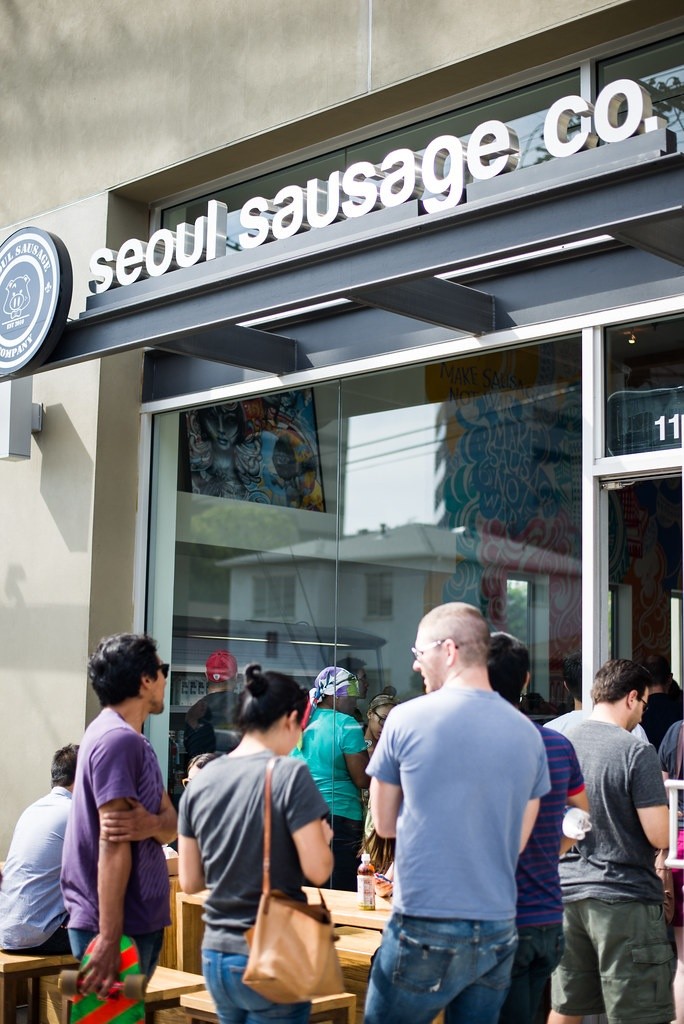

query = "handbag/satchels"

[655,847,675,920]
[242,892,348,1004]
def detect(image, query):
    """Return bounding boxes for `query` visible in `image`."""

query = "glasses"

[182,778,190,788]
[374,710,386,726]
[158,664,170,678]
[637,696,650,712]
[411,641,459,662]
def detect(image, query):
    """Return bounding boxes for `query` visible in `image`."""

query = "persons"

[558,660,679,1024]
[640,655,684,1024]
[356,829,395,897]
[560,647,649,742]
[337,657,369,736]
[182,648,246,769]
[524,693,557,725]
[182,753,219,789]
[176,661,338,1024]
[363,686,401,757]
[361,601,551,1024]
[61,633,176,1000]
[289,665,371,892]
[485,631,590,1024]
[0,745,80,956]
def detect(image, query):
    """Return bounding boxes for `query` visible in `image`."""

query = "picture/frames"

[184,387,327,515]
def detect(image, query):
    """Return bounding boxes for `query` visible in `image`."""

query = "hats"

[206,650,237,682]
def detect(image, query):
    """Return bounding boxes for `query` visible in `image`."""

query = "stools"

[0,943,356,1024]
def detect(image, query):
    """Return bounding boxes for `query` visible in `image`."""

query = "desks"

[176,884,395,975]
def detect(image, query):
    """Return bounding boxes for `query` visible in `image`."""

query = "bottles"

[191,682,196,694]
[357,854,375,910]
[199,682,204,694]
[561,805,592,840]
[182,682,188,694]
